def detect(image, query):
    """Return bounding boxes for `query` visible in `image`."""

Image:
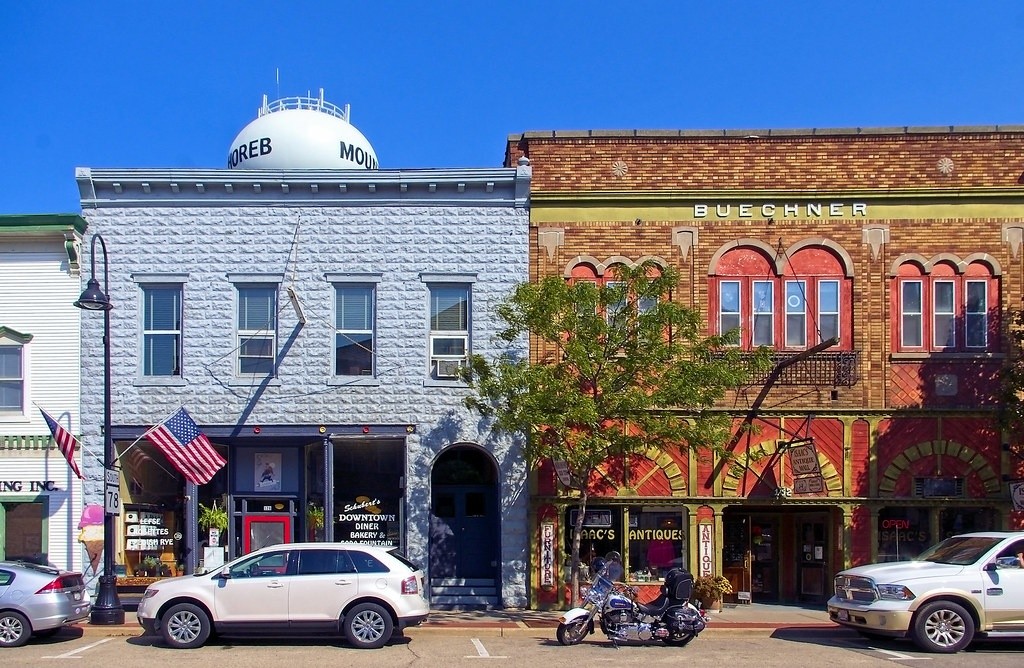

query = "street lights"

[73,233,129,627]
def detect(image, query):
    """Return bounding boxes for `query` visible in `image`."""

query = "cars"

[135,541,430,650]
[0,558,93,648]
[827,531,1024,653]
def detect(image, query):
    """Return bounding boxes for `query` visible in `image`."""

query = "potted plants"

[197,502,229,547]
[307,506,325,542]
[694,574,732,610]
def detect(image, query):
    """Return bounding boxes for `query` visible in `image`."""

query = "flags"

[145,408,228,486]
[37,406,86,480]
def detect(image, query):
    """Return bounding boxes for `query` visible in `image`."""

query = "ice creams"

[77,505,104,573]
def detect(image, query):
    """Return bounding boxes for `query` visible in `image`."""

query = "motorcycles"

[555,560,712,651]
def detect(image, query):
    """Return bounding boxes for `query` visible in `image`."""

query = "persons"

[1013,545,1024,569]
[608,556,624,583]
